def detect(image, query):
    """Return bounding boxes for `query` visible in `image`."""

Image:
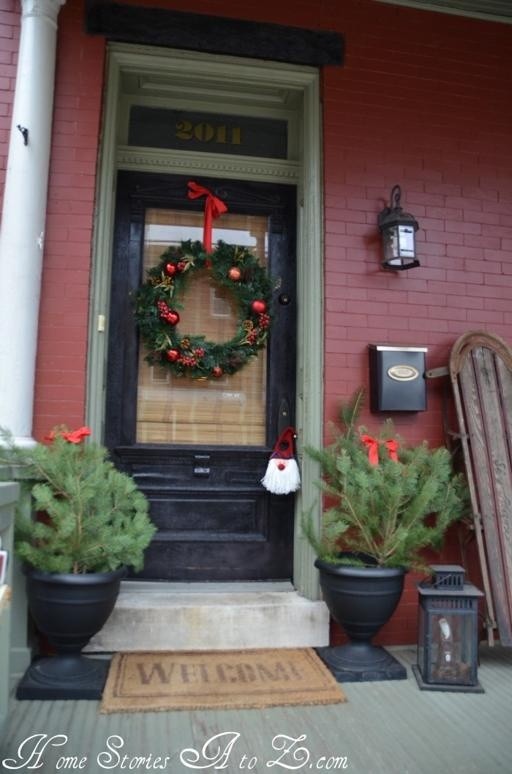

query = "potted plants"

[0,421,160,699]
[299,379,474,687]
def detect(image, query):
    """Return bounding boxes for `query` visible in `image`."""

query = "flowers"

[133,237,277,387]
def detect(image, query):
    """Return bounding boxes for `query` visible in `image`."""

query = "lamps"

[412,563,484,695]
[379,182,421,273]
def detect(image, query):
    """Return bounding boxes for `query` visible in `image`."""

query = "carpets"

[100,643,346,716]
